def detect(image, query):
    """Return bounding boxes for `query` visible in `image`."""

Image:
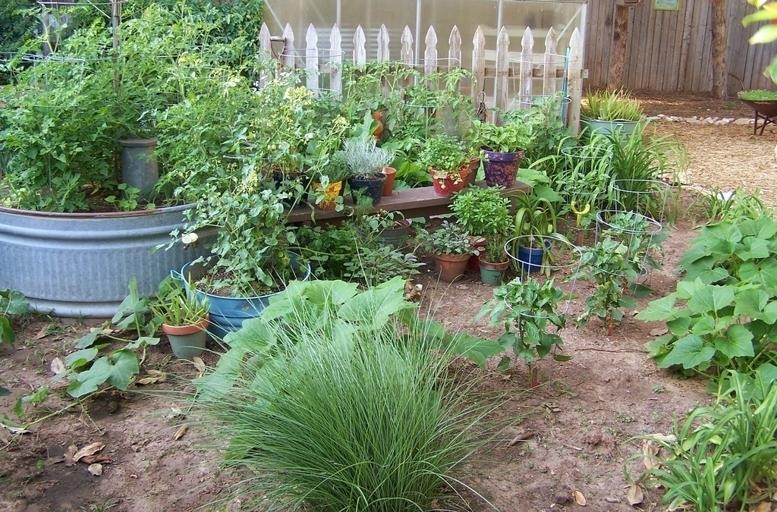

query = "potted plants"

[147,83,310,361]
[310,84,647,286]
